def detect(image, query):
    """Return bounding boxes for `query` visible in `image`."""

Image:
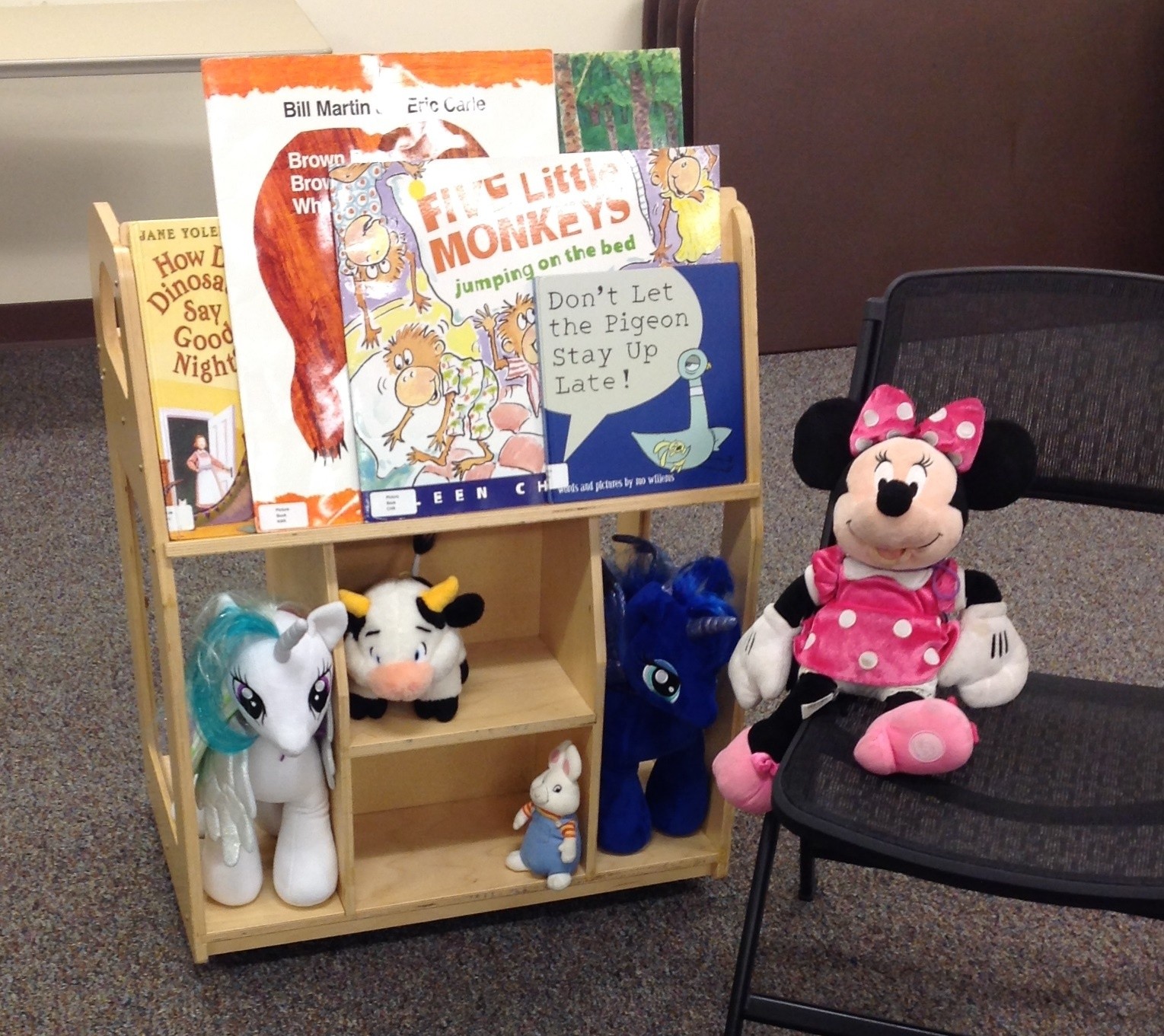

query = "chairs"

[724,266,1164,1036]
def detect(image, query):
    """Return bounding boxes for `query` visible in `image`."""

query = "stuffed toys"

[170,594,349,908]
[710,384,1038,815]
[504,739,582,891]
[595,534,743,856]
[339,532,486,723]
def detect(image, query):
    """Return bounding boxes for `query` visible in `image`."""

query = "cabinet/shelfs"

[86,187,768,968]
